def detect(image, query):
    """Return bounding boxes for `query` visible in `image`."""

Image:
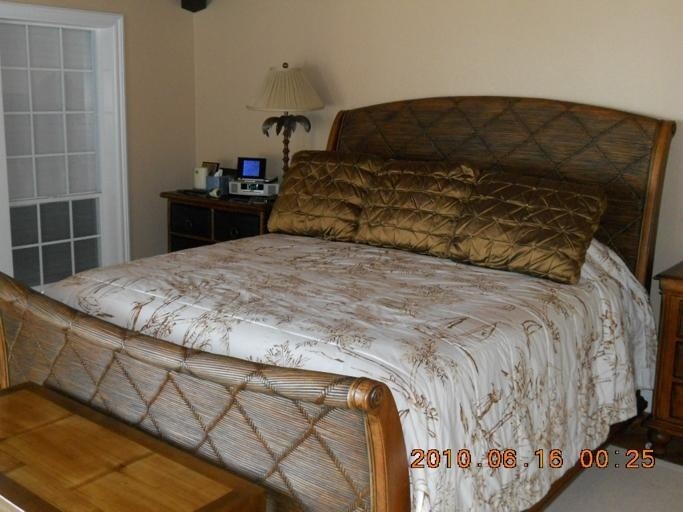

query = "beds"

[0,96,677,512]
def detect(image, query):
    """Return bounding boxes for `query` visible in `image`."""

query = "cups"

[193,167,208,190]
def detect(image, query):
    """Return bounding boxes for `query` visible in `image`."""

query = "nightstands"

[158,191,272,254]
[641,261,683,455]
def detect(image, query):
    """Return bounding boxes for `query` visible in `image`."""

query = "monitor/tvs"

[237,157,267,180]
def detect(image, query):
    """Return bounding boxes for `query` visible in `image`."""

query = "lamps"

[246,62,324,174]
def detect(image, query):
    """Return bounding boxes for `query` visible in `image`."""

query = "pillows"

[355,157,481,257]
[449,165,610,285]
[268,150,390,243]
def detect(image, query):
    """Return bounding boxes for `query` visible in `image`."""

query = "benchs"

[0,381,269,512]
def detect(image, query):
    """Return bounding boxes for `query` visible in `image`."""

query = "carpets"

[540,444,683,512]
